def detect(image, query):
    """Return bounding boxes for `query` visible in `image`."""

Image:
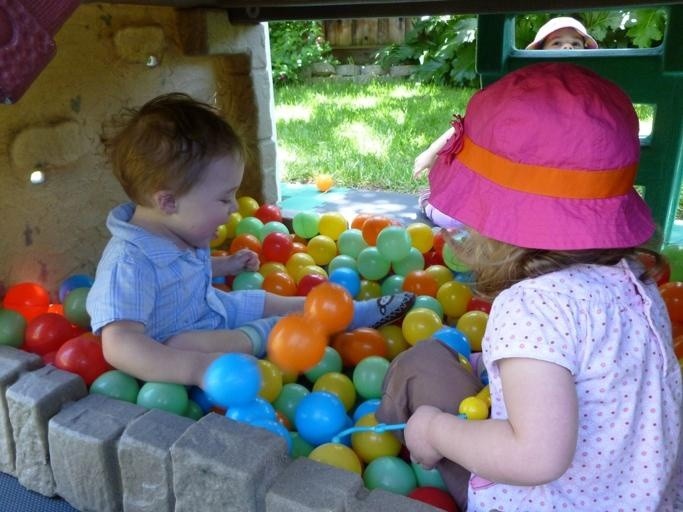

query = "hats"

[429,61,654,250]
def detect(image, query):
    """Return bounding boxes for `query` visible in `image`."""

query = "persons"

[375,63,683,512]
[84,91,416,392]
